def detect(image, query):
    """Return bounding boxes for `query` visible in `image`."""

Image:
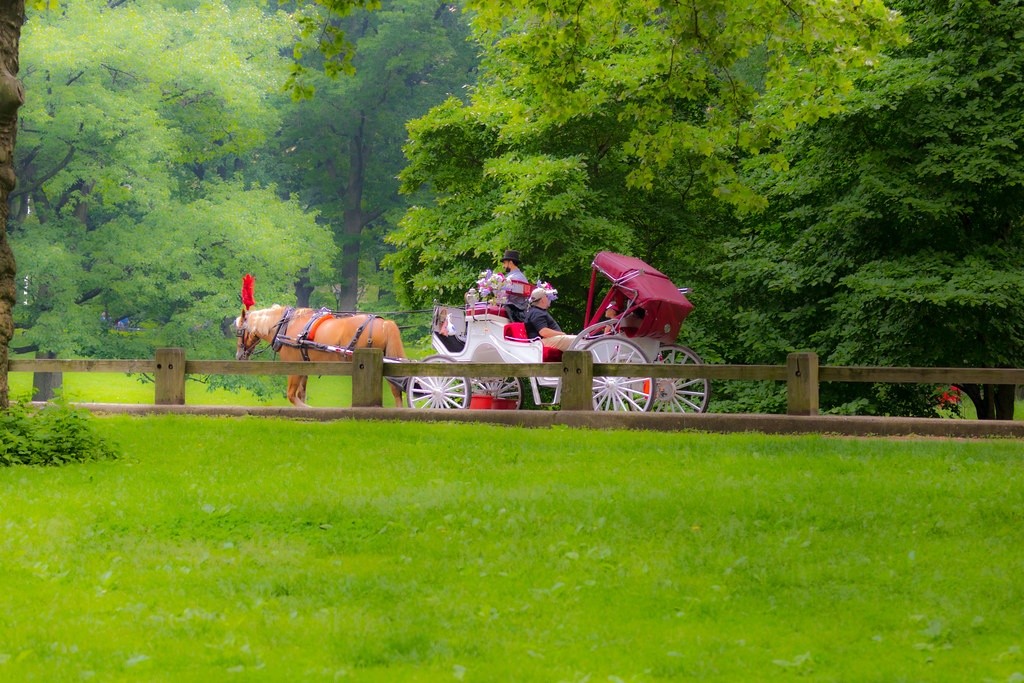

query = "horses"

[235,300,410,410]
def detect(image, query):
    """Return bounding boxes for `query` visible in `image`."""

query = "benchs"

[466,279,537,319]
[503,322,563,363]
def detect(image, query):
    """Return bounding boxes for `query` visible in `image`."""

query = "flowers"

[476,270,559,305]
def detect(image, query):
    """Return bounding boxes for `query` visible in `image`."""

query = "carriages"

[229,250,714,413]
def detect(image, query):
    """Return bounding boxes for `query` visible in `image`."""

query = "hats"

[527,288,546,303]
[606,301,621,312]
[500,250,522,264]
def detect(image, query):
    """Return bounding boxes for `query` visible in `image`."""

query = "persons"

[604,300,644,338]
[524,289,583,351]
[500,250,528,303]
